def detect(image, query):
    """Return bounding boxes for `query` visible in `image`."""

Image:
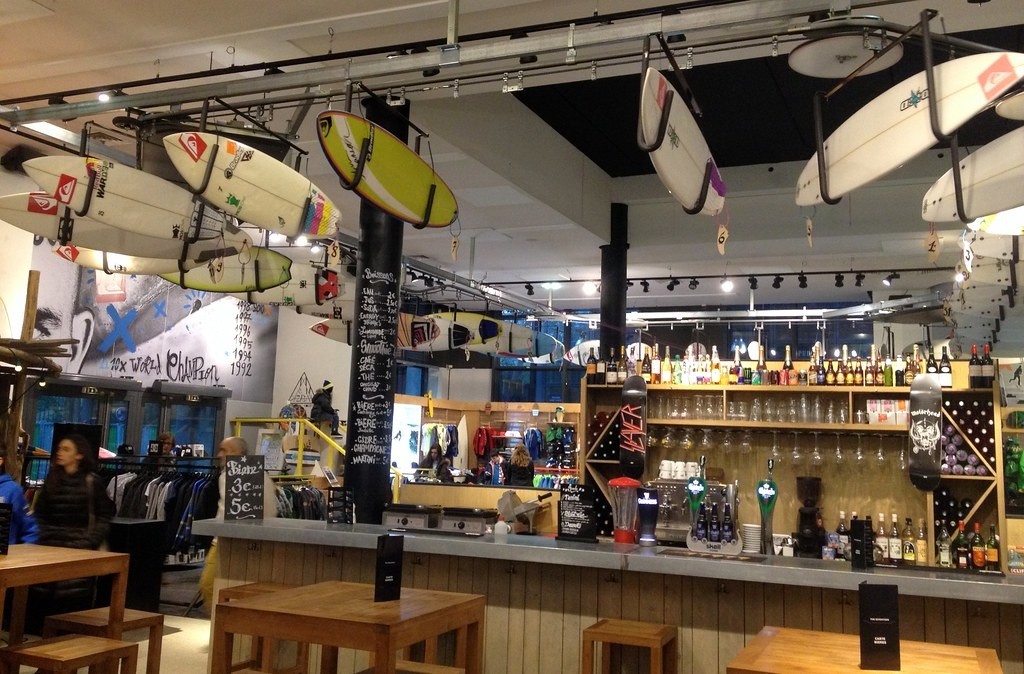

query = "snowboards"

[907,373,943,492]
[617,374,648,479]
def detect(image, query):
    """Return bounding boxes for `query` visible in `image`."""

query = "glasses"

[164,442,171,444]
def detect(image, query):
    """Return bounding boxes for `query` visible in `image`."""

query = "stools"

[0,634,138,674]
[580,618,678,674]
[45,606,165,674]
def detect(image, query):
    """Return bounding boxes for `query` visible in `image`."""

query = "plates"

[743,524,761,553]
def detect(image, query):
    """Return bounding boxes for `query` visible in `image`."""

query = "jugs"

[495,516,511,534]
[854,410,869,424]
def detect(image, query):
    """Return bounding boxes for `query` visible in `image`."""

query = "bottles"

[588,344,952,387]
[1003,437,1024,495]
[697,502,734,542]
[835,510,928,566]
[969,344,993,388]
[935,486,1000,571]
[946,400,996,464]
[587,410,620,458]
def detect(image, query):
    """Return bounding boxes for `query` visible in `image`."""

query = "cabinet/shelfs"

[645,382,1004,576]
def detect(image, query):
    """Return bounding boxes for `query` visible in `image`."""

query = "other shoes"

[332,432,342,437]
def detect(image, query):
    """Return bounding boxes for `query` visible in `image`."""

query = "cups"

[649,393,848,422]
[828,532,839,548]
[658,460,706,480]
[822,546,834,560]
[773,534,792,545]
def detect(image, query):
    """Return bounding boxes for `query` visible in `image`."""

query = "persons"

[199,436,277,616]
[0,436,112,634]
[413,443,450,484]
[465,444,534,487]
[310,380,344,438]
[143,431,177,471]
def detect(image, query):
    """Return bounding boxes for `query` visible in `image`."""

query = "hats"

[323,381,333,389]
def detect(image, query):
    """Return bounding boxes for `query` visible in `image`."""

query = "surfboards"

[640,67,728,217]
[902,205,1024,408]
[0,153,294,295]
[313,109,461,229]
[793,51,1024,208]
[564,339,600,366]
[162,131,343,240]
[308,308,566,365]
[223,261,357,321]
[920,125,1024,223]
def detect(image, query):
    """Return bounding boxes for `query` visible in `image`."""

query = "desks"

[726,624,1002,674]
[0,544,130,674]
[210,577,486,674]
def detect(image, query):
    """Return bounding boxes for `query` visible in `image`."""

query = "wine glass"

[647,425,907,472]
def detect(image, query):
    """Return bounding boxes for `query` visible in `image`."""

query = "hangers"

[94,459,219,483]
[277,480,314,490]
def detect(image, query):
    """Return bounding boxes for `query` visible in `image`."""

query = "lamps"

[525,282,534,295]
[625,269,901,292]
[406,269,445,289]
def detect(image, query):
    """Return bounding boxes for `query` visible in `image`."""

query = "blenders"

[797,477,826,556]
